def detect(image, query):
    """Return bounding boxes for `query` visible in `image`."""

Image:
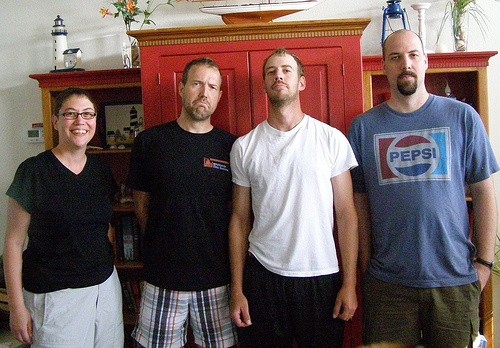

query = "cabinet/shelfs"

[29,18,498,348]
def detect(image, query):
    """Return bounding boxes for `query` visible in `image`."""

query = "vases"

[121,44,141,69]
[452,9,469,51]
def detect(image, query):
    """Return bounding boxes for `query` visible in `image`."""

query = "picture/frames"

[98,100,145,149]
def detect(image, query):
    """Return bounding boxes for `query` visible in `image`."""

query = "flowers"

[99,0,175,47]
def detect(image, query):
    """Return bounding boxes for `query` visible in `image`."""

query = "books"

[110,218,142,263]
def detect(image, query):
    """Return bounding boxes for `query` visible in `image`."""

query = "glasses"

[57,111,97,120]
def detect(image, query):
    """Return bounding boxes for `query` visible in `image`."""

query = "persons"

[230,49,358,348]
[346,30,500,348]
[4,87,125,348]
[121,57,253,348]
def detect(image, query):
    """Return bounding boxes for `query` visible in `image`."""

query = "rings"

[351,315,354,316]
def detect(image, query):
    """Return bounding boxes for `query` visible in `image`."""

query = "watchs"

[475,257,494,271]
